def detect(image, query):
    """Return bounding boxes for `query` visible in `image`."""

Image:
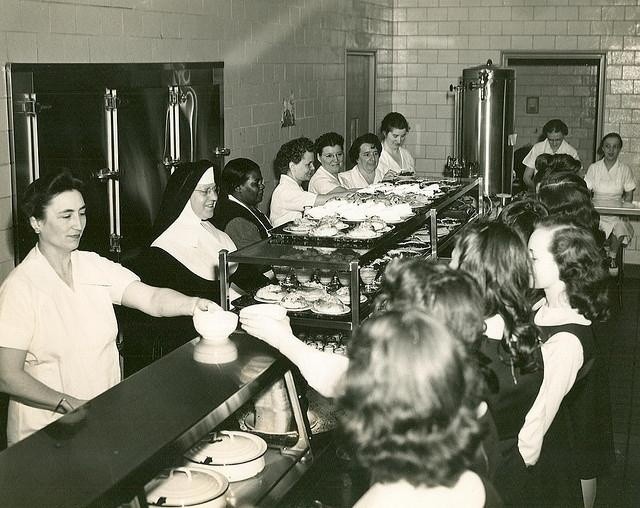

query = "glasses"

[194,185,220,194]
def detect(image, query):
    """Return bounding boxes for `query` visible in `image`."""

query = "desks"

[590,194,638,220]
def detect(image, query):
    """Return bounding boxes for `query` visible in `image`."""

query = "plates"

[364,218,458,294]
[286,175,461,239]
[246,407,319,436]
[255,281,368,317]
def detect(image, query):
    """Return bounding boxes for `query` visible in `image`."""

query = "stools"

[599,237,628,311]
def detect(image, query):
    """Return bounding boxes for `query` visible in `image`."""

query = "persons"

[242,120,635,507]
[1,112,416,457]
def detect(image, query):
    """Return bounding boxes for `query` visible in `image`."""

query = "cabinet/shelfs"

[216,171,490,339]
[1,330,359,508]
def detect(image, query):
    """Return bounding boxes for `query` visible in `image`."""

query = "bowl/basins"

[192,309,244,342]
[139,429,270,507]
[193,339,238,368]
